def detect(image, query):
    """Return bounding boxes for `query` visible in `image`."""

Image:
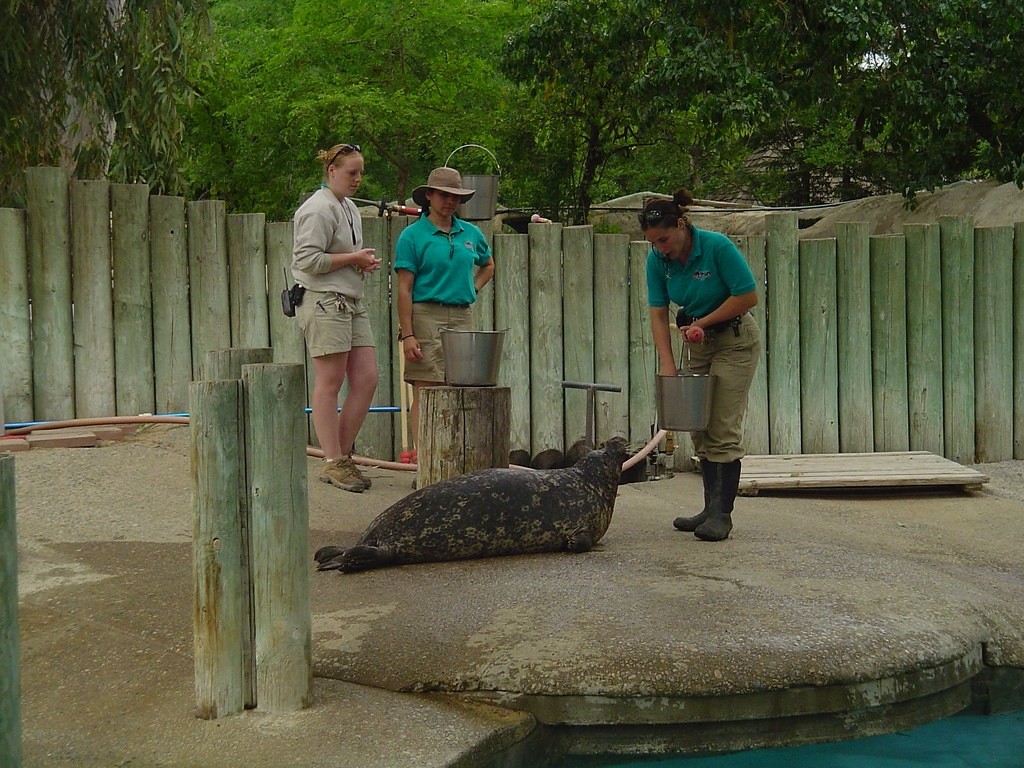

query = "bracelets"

[401,335,415,342]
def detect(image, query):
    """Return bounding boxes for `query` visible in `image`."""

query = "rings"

[417,349,421,350]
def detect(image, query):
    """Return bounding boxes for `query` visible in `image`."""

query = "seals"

[313,436,632,575]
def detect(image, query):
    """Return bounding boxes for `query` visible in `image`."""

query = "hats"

[412,167,475,206]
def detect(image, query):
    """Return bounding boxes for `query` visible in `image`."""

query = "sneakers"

[318,450,372,493]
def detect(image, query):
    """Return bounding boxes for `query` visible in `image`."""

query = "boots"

[694,460,741,541]
[673,459,717,532]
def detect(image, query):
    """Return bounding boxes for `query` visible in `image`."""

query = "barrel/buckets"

[444,144,501,220]
[438,326,511,386]
[655,340,718,431]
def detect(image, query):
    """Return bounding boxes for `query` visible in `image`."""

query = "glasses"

[448,234,456,260]
[638,209,680,226]
[326,144,361,172]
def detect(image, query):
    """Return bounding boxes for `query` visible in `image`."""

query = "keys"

[335,292,347,312]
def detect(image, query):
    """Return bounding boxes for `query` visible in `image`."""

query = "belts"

[425,299,469,309]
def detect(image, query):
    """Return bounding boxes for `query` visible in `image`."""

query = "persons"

[638,189,761,541]
[290,144,383,492]
[393,167,494,446]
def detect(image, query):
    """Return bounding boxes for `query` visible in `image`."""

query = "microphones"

[652,244,668,263]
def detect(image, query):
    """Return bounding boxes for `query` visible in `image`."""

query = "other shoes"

[411,475,419,490]
